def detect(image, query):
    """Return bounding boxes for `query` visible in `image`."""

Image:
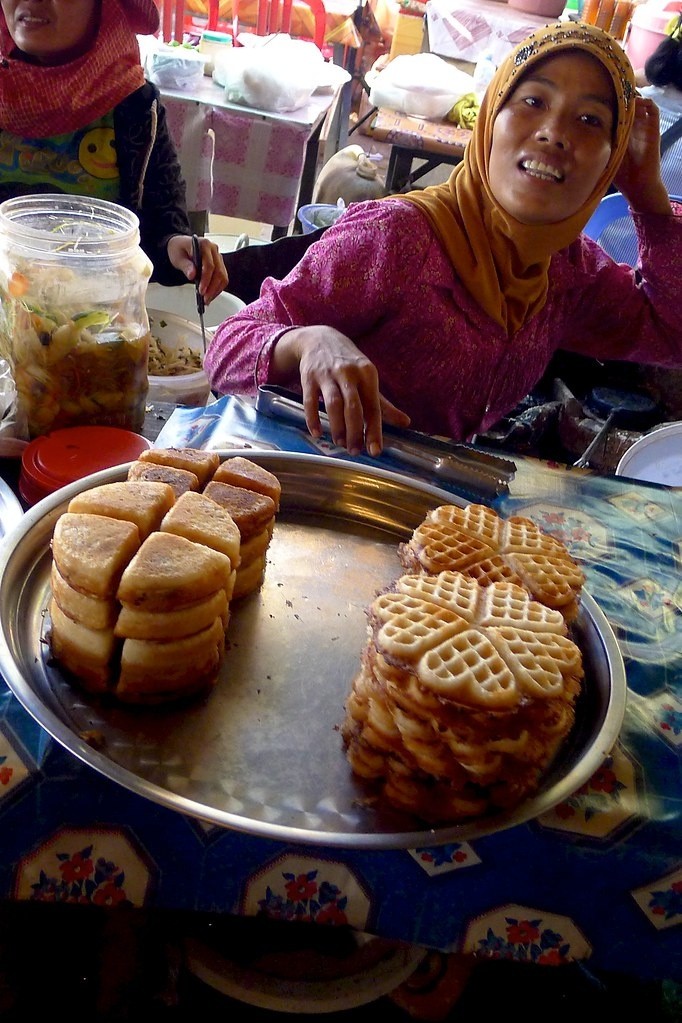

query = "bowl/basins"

[297,204,347,234]
[381,70,474,122]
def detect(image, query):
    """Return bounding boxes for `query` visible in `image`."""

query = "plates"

[212,47,352,90]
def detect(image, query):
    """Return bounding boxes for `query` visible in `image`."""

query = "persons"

[0,0,229,307]
[203,21,682,459]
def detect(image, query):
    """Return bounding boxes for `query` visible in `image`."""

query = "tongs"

[255,384,517,501]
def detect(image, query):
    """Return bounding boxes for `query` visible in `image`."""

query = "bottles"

[199,31,234,76]
[473,54,496,88]
[581,0,632,40]
[1,194,151,453]
[389,10,424,63]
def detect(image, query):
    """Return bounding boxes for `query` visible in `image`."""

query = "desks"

[420,0,631,77]
[158,74,345,242]
[371,105,473,195]
[0,394,682,1023]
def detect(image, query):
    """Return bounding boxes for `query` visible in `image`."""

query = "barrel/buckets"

[145,282,248,335]
[310,144,385,207]
[145,307,216,408]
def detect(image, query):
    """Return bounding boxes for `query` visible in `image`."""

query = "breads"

[50,449,281,707]
[340,502,585,824]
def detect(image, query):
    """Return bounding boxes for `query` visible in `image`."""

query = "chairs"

[582,192,682,268]
[153,0,326,51]
